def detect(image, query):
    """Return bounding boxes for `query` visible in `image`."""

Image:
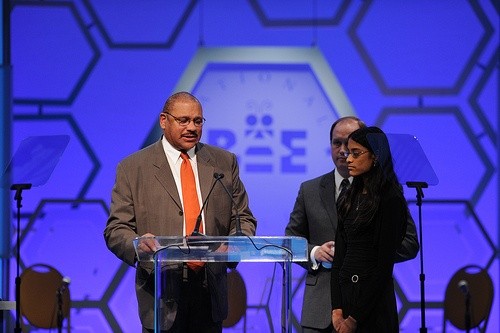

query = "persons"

[281,116,418,332]
[105,90,257,333]
[330,126,407,333]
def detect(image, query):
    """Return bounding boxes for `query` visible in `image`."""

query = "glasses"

[163,112,205,126]
[344,150,369,158]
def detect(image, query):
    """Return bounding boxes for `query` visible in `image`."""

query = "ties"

[179,153,206,274]
[337,179,350,211]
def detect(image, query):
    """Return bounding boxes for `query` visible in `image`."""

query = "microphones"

[194,173,224,232]
[213,172,242,233]
